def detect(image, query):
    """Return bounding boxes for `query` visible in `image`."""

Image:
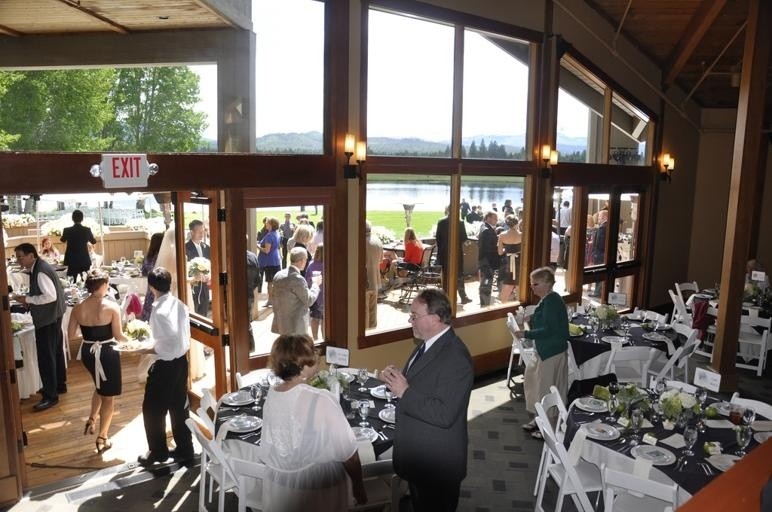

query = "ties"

[407,341,427,375]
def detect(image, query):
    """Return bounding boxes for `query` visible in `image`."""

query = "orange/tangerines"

[704,442,716,454]
[705,407,718,417]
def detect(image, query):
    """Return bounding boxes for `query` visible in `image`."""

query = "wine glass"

[10,247,146,306]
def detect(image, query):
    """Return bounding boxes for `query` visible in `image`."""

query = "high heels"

[84,418,96,437]
[91,434,113,455]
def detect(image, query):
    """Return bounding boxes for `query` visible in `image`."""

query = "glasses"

[530,280,542,288]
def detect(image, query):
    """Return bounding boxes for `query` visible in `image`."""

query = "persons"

[60,209,97,283]
[515,267,568,439]
[13,243,67,411]
[556,201,609,297]
[435,198,523,308]
[390,227,424,279]
[365,220,383,330]
[382,250,398,260]
[134,267,194,466]
[246,212,325,353]
[383,288,475,512]
[141,233,164,323]
[260,333,368,512]
[40,239,59,257]
[745,258,769,295]
[186,220,210,318]
[68,270,131,452]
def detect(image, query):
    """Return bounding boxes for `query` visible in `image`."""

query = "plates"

[220,368,395,444]
[575,380,771,474]
[563,302,672,345]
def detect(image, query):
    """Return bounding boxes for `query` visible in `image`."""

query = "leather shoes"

[461,296,472,305]
[31,396,61,410]
[137,450,169,464]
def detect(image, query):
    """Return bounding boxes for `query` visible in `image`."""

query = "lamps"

[660,153,677,183]
[343,133,367,181]
[536,144,560,180]
